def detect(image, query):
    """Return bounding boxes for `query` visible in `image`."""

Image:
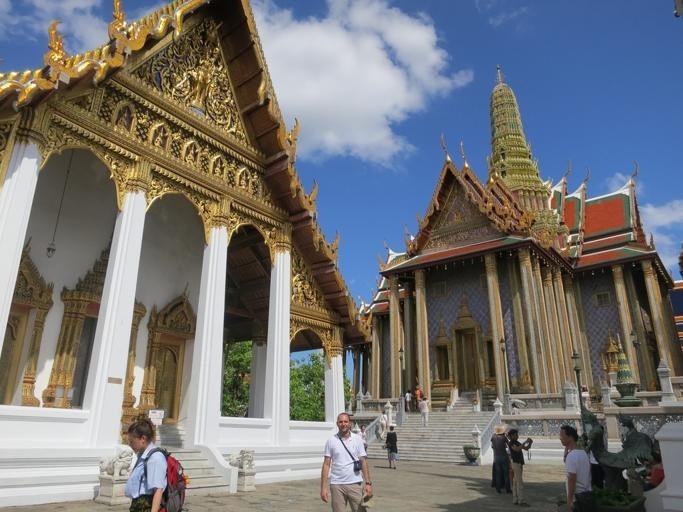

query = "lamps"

[45,148,83,258]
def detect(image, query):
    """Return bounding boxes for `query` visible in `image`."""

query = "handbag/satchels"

[354,461,361,471]
[575,491,597,511]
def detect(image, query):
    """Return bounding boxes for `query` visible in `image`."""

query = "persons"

[124,413,168,512]
[320,410,397,512]
[405,385,431,426]
[491,414,608,512]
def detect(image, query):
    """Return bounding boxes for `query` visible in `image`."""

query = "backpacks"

[144,448,189,512]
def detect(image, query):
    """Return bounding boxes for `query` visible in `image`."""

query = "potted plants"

[559,487,646,512]
[462,444,480,467]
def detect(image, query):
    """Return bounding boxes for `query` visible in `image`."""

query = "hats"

[494,423,510,434]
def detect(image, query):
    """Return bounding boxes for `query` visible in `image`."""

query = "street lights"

[571,348,588,448]
